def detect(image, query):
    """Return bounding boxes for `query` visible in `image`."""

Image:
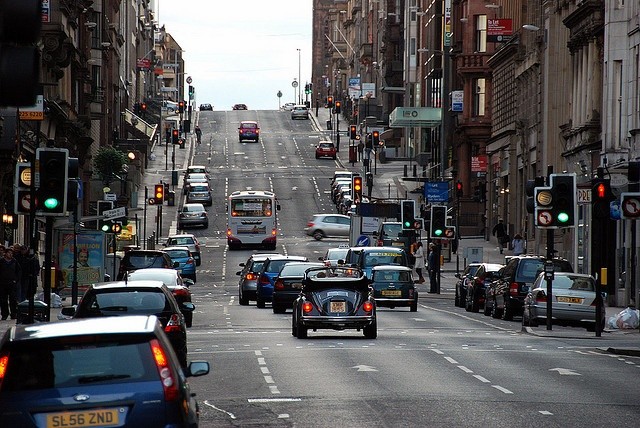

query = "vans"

[358,248,409,280]
[304,213,350,240]
[338,247,362,266]
[238,121,260,143]
[372,221,420,265]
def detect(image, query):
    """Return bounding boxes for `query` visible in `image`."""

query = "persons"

[338,259,344,265]
[366,140,373,160]
[317,272,326,278]
[318,257,323,262]
[351,264,357,268]
[413,242,425,284]
[511,234,525,255]
[20,246,40,302]
[325,262,331,266]
[0,245,6,257]
[493,220,506,254]
[78,249,89,267]
[427,243,438,293]
[0,249,21,320]
[195,126,201,143]
[358,140,364,162]
[12,244,22,265]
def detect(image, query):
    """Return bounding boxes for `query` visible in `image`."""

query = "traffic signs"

[357,236,369,246]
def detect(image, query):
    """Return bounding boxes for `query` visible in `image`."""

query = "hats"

[498,218,503,221]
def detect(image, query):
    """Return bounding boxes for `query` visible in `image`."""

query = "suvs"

[257,256,307,308]
[290,105,309,120]
[162,234,200,266]
[186,183,212,205]
[183,165,206,177]
[0,315,210,428]
[315,141,337,160]
[62,281,195,367]
[484,254,574,321]
[291,266,377,339]
[235,254,284,305]
[117,249,180,280]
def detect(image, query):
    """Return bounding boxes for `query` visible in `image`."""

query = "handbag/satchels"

[503,235,509,242]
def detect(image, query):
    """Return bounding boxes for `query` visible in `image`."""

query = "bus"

[227,190,280,250]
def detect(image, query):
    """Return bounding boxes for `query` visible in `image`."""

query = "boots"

[418,278,422,284]
[421,277,425,282]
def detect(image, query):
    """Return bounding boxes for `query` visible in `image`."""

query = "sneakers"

[500,250,502,254]
[426,291,434,293]
[1,313,9,319]
[10,313,16,319]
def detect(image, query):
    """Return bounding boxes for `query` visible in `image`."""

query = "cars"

[522,271,605,332]
[318,244,350,264]
[178,203,208,229]
[232,104,248,110]
[368,264,417,311]
[183,173,209,195]
[465,263,505,316]
[454,263,481,311]
[329,171,353,214]
[198,104,214,111]
[121,268,195,327]
[160,247,196,282]
[273,262,325,313]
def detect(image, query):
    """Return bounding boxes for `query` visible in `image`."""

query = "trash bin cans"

[327,120,331,130]
[180,138,185,148]
[305,102,310,107]
[167,192,175,206]
[15,300,48,324]
[350,146,356,162]
[172,170,178,185]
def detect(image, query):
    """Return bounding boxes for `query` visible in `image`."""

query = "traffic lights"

[305,85,309,94]
[351,126,356,139]
[139,102,146,113]
[353,176,363,204]
[179,102,183,113]
[189,86,192,96]
[401,200,424,231]
[336,102,341,113]
[309,83,312,93]
[173,130,178,144]
[328,96,333,107]
[34,148,69,217]
[183,100,187,110]
[430,205,455,239]
[96,201,122,233]
[67,157,79,206]
[155,185,163,204]
[456,182,463,198]
[591,178,611,220]
[552,176,574,226]
[373,131,379,145]
